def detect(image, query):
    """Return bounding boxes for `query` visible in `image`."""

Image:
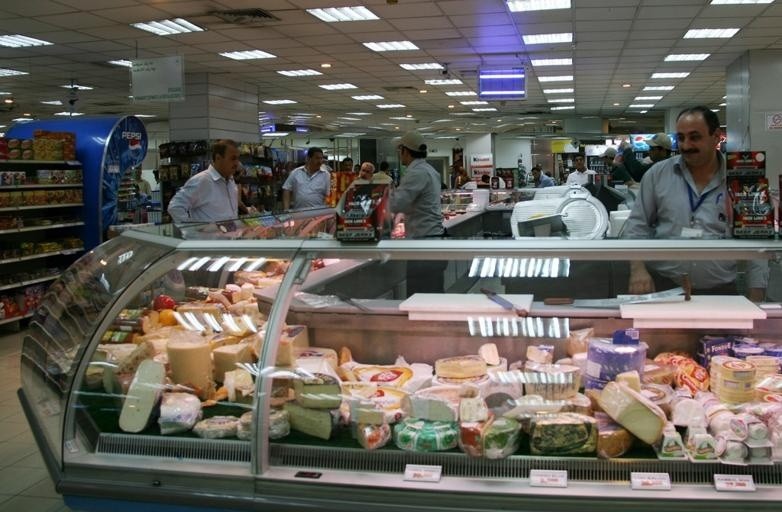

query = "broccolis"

[629,132,679,161]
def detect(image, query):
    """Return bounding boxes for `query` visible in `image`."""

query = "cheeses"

[118,271,667,460]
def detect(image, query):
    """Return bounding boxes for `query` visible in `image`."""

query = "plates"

[161,146,299,219]
[0,155,86,329]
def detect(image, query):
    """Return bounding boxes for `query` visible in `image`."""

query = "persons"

[535,164,544,174]
[165,137,241,223]
[341,157,353,172]
[531,167,554,188]
[280,146,330,211]
[479,175,490,184]
[134,184,149,224]
[388,130,449,297]
[374,161,393,182]
[357,162,374,184]
[617,103,772,302]
[564,131,674,188]
[134,162,152,196]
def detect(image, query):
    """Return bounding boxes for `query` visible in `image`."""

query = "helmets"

[473,190,489,209]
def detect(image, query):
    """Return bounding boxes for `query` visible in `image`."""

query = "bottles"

[598,148,617,158]
[392,130,427,152]
[644,134,672,150]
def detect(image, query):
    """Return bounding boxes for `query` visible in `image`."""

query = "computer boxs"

[441,65,449,75]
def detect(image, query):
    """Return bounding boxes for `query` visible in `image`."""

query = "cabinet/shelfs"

[117,201,150,224]
[0,155,86,329]
[161,146,299,219]
[19,194,782,512]
